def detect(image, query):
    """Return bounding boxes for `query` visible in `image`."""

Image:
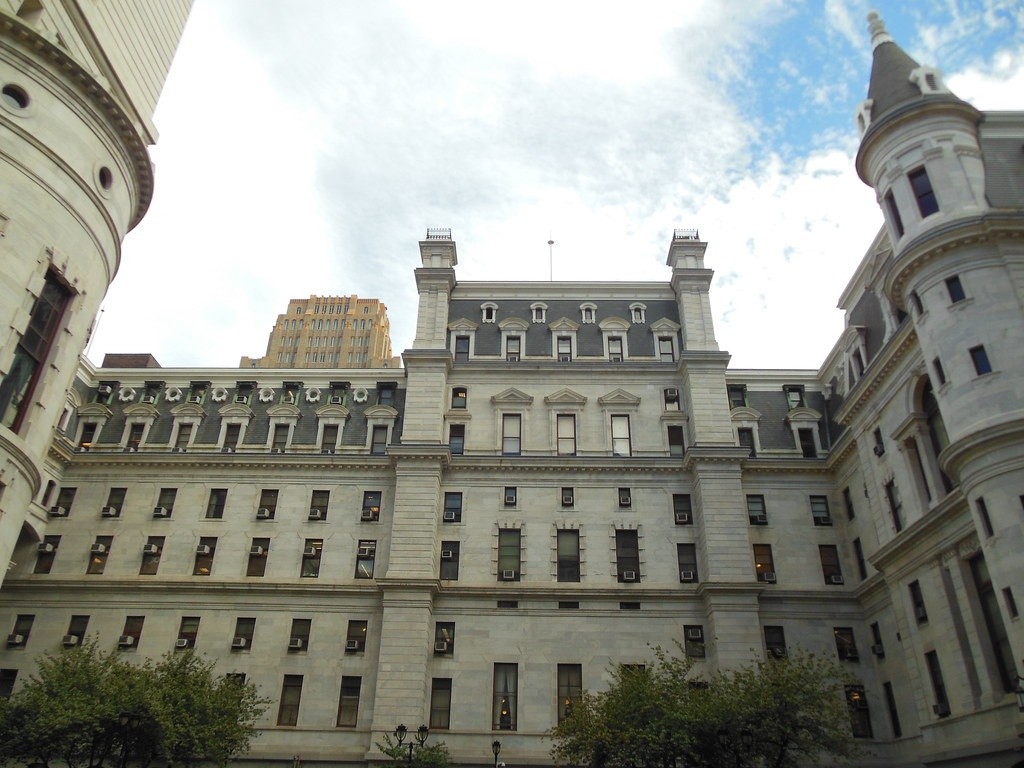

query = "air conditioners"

[755,514,768,525]
[843,648,859,658]
[761,573,775,582]
[620,496,630,506]
[441,550,452,558]
[434,641,448,652]
[666,388,678,398]
[818,516,833,526]
[444,512,455,521]
[870,644,883,655]
[5,386,374,650]
[563,496,573,505]
[623,570,635,583]
[688,629,702,641]
[503,570,514,581]
[508,356,621,362]
[932,703,948,715]
[828,576,844,585]
[776,648,786,657]
[505,496,515,505]
[681,571,693,582]
[873,444,884,455]
[675,513,688,524]
[915,607,926,619]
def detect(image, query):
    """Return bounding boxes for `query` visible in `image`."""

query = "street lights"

[393,724,429,768]
[492,740,501,768]
